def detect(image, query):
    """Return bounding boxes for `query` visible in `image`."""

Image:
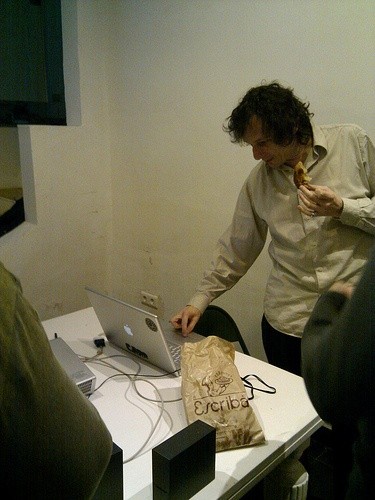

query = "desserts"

[294,162,312,190]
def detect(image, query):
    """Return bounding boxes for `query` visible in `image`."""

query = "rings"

[308,212,316,218]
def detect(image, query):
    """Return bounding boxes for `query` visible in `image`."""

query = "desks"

[40,306,326,500]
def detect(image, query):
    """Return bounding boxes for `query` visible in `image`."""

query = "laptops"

[85,289,208,377]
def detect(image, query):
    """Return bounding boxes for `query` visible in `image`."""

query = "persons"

[300,247,375,500]
[169,79,375,500]
[0,259,112,500]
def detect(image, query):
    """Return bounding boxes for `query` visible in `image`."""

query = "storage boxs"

[151,420,216,500]
